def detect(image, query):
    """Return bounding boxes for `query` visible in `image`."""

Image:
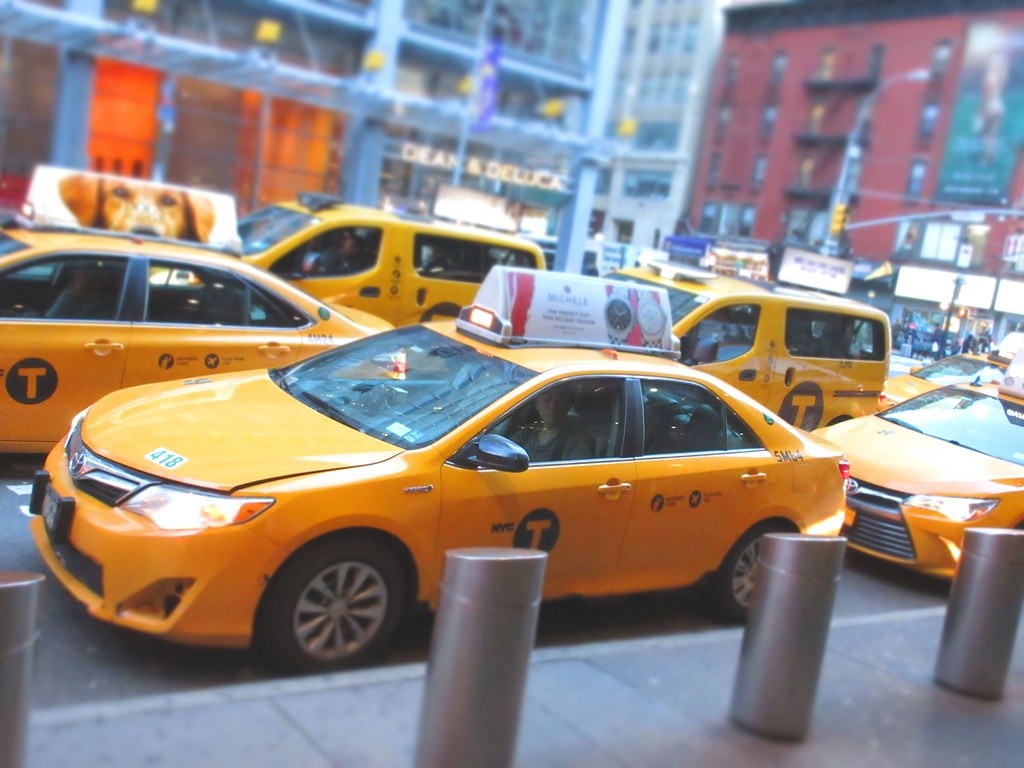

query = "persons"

[47,261,116,319]
[310,231,369,279]
[509,378,596,463]
[890,318,992,365]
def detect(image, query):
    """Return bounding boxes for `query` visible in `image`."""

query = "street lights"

[825,66,931,256]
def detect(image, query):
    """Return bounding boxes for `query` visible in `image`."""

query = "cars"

[17,266,849,671]
[0,221,396,456]
[876,329,1024,413]
[811,349,1024,585]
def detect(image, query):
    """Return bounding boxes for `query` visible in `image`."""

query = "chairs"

[655,403,724,455]
[643,395,679,446]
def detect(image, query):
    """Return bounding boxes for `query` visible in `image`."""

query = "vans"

[218,196,552,337]
[596,265,895,437]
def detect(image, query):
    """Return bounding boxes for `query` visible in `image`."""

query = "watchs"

[633,289,667,350]
[603,284,638,347]
